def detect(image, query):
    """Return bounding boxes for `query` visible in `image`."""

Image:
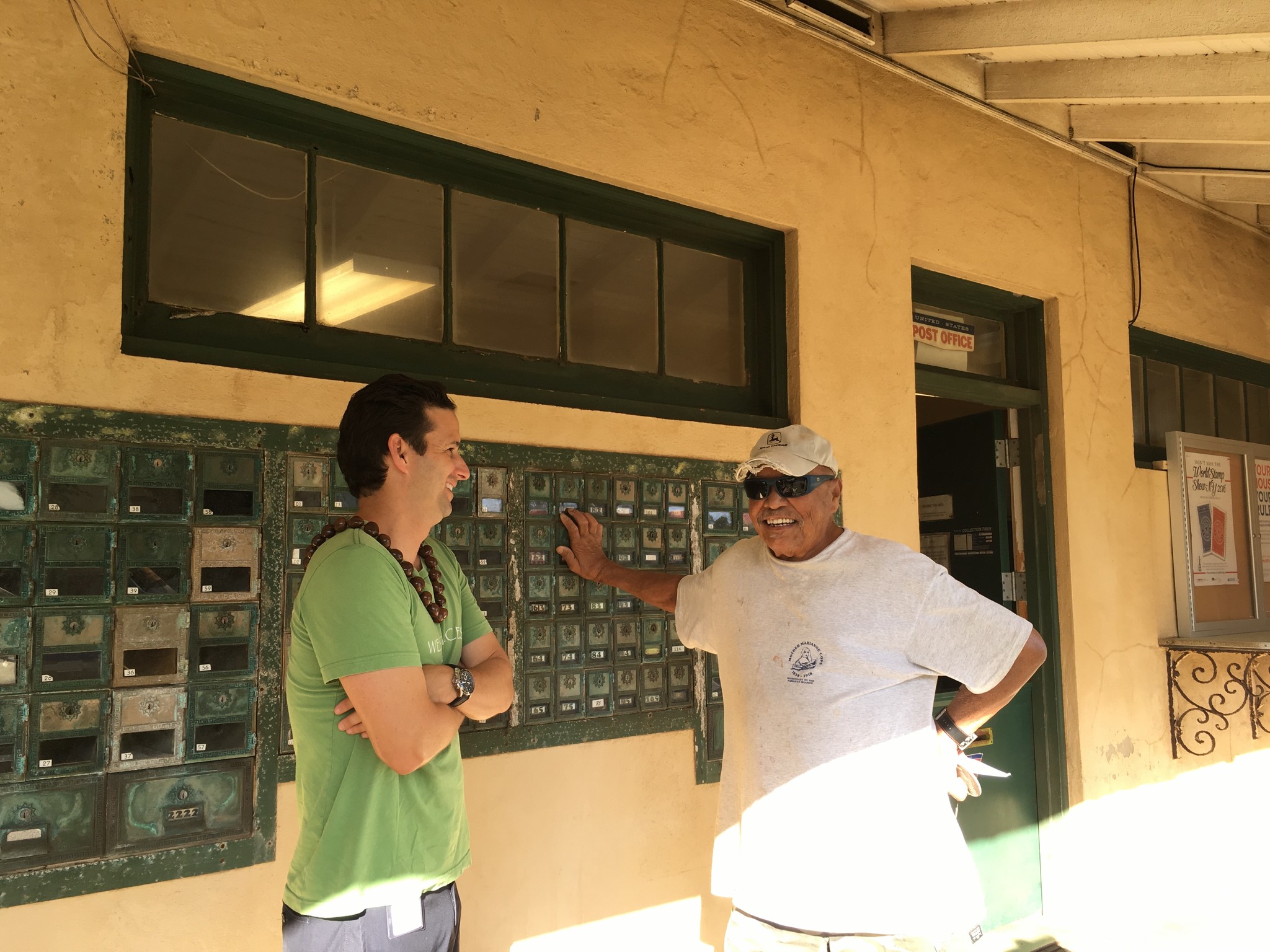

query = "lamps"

[239,201,439,326]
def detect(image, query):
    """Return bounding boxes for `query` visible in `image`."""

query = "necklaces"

[304,516,449,624]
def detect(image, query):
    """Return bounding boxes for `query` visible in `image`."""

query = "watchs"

[444,663,475,708]
[934,707,978,751]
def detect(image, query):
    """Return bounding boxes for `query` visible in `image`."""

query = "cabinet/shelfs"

[0,399,844,908]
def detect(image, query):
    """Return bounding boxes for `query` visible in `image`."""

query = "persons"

[556,423,1049,952]
[282,373,513,952]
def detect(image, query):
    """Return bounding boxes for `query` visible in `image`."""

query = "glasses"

[743,475,835,500]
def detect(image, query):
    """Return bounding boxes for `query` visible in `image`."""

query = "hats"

[735,424,838,482]
